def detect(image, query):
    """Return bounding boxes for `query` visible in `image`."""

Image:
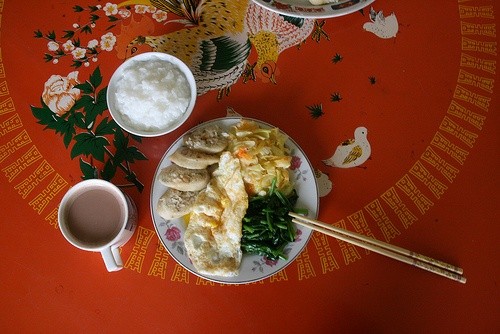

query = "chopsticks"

[287,211,468,285]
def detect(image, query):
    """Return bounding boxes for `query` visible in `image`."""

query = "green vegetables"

[240,180,309,262]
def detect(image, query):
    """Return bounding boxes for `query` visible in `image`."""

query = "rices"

[114,60,191,132]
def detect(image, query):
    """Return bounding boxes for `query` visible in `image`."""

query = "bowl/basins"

[106,52,197,137]
[252,0,375,18]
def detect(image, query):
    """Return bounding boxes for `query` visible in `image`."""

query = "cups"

[57,179,139,273]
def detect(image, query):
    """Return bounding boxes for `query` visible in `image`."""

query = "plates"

[149,117,320,285]
